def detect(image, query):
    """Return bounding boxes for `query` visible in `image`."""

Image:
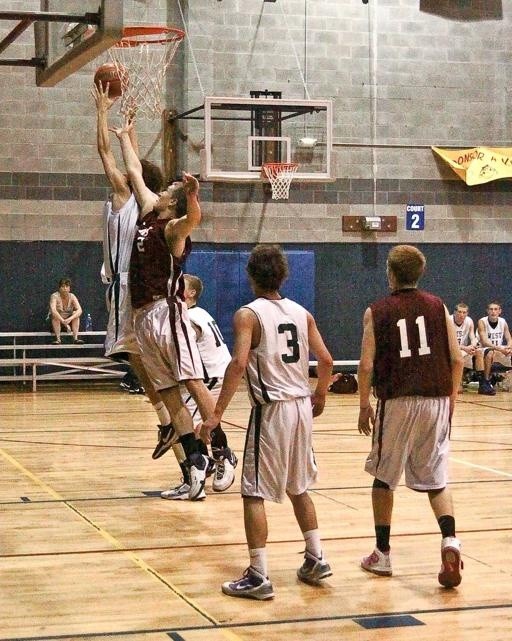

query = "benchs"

[0,329,133,393]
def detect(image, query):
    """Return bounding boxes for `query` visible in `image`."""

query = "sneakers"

[128,387,145,394]
[72,339,84,344]
[438,537,463,588]
[160,482,206,500]
[360,549,393,576]
[52,339,61,344]
[119,382,130,390]
[212,447,238,492]
[296,550,333,584]
[152,422,179,460]
[477,384,496,395]
[188,453,213,501]
[221,565,275,600]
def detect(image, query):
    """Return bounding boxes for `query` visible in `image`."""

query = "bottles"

[86,313,92,332]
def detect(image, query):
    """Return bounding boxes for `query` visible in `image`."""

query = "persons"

[88,78,183,460]
[161,271,230,502]
[44,274,83,345]
[451,303,495,397]
[475,300,512,390]
[109,112,239,503]
[358,245,465,588]
[196,240,333,600]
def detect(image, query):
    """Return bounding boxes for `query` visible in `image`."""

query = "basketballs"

[93,62,129,98]
